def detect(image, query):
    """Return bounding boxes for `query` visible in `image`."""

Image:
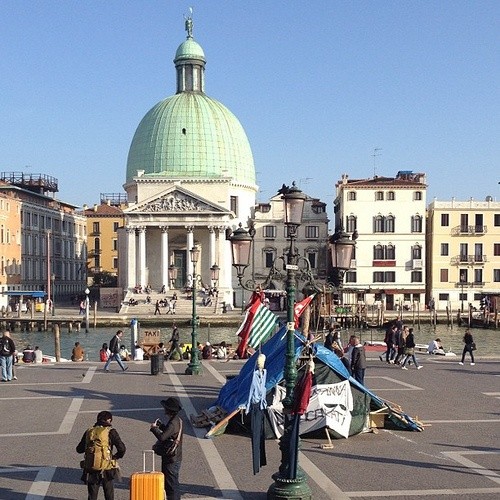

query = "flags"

[236,302,280,348]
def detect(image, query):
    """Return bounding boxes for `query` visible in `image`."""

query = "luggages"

[130,450,166,500]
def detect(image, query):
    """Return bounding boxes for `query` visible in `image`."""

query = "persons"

[134,284,152,294]
[164,323,184,361]
[154,300,161,315]
[78,300,84,315]
[0,331,17,382]
[379,321,423,371]
[145,296,152,305]
[427,338,443,355]
[119,345,129,361]
[134,345,144,361]
[129,297,136,306]
[100,343,111,362]
[172,341,227,360]
[25,299,32,313]
[70,342,84,362]
[322,328,366,386]
[104,330,128,372]
[458,329,475,366]
[76,411,125,500]
[159,285,177,315]
[150,398,183,500]
[22,345,43,364]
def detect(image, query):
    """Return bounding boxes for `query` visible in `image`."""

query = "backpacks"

[84,426,116,478]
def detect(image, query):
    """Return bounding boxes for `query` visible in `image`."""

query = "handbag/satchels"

[467,342,476,352]
[153,439,176,460]
[405,348,413,355]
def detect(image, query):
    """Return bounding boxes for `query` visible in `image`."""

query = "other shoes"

[470,363,475,365]
[458,362,464,365]
[410,362,414,366]
[402,366,408,370]
[417,365,423,369]
[379,355,401,366]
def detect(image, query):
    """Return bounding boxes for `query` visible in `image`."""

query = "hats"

[198,345,204,351]
[179,343,185,347]
[206,342,210,346]
[435,338,441,342]
[160,397,183,411]
